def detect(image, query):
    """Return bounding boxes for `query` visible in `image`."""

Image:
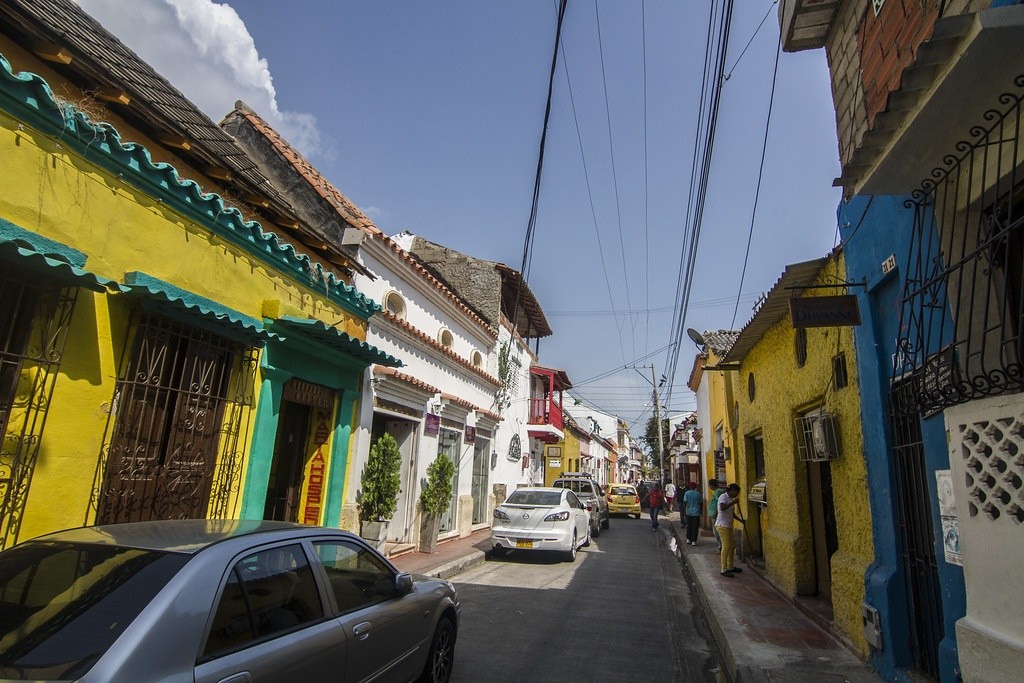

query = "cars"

[636,481,659,497]
[603,483,641,519]
[0,519,463,682]
[491,487,593,562]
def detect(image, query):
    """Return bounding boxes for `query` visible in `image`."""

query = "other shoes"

[681,525,684,528]
[692,541,696,546]
[652,528,656,532]
[721,570,733,577]
[730,567,742,572]
[686,539,691,543]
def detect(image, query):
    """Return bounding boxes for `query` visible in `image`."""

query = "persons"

[715,483,746,578]
[683,482,703,547]
[624,479,649,512]
[664,479,676,513]
[676,479,690,527]
[708,478,724,553]
[644,483,665,532]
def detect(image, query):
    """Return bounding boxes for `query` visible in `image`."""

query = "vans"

[552,471,610,536]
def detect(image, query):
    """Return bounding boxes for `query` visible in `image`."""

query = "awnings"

[719,256,824,365]
[0,238,131,297]
[280,315,408,370]
[123,284,286,345]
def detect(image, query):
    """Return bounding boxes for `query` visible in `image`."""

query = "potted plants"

[359,432,402,556]
[420,453,455,554]
